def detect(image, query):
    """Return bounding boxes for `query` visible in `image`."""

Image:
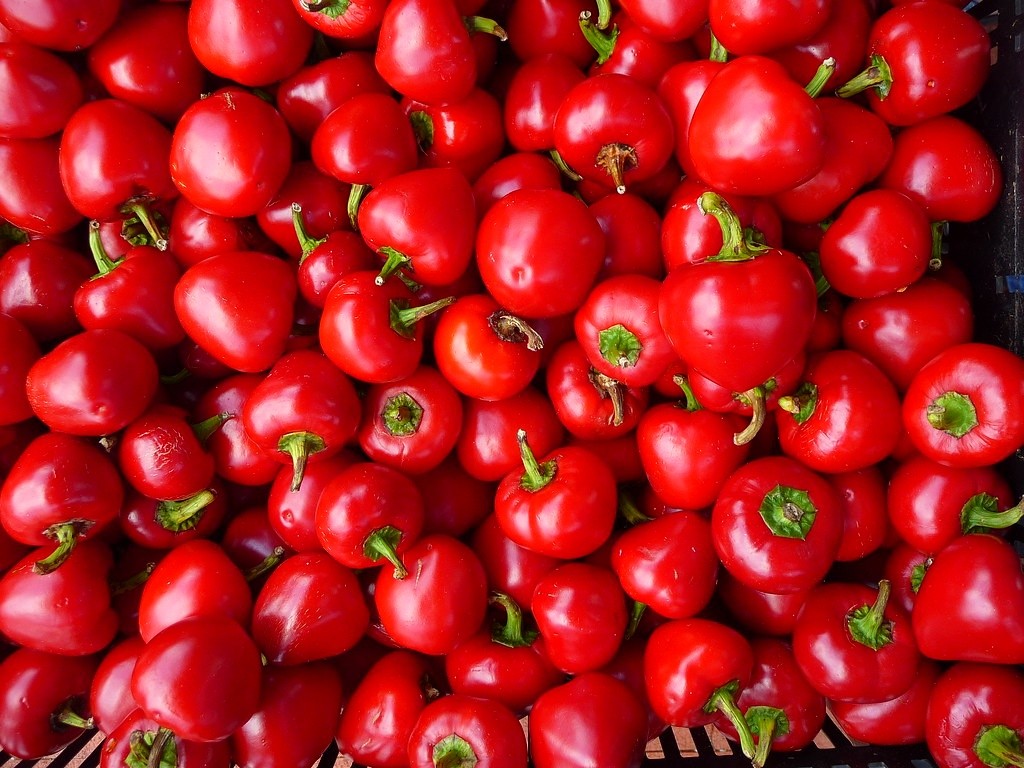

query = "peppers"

[0,0,1024,768]
